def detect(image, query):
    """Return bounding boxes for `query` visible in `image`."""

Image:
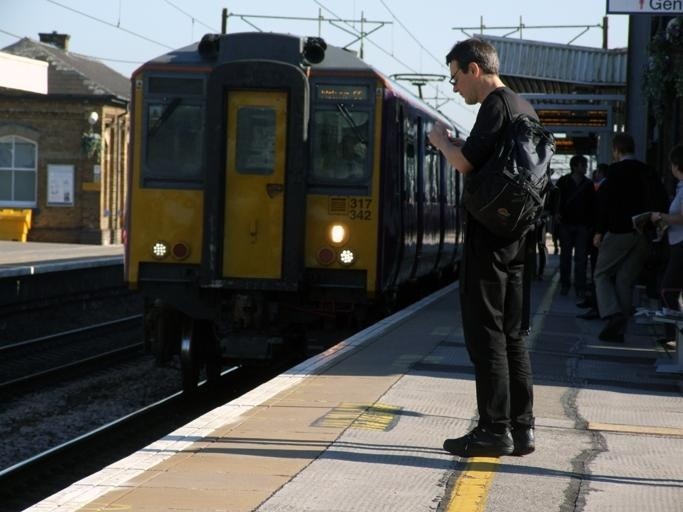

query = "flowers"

[638,17,683,129]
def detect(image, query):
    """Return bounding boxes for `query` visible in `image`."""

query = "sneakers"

[442,427,515,458]
[510,423,535,457]
[560,283,665,344]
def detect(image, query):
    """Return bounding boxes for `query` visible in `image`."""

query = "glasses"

[447,68,460,87]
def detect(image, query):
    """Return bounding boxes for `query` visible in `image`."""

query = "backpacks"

[464,89,556,245]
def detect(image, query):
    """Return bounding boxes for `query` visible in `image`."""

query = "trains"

[118,10,478,407]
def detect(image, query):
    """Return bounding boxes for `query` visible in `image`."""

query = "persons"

[523,130,682,345]
[428,37,557,457]
